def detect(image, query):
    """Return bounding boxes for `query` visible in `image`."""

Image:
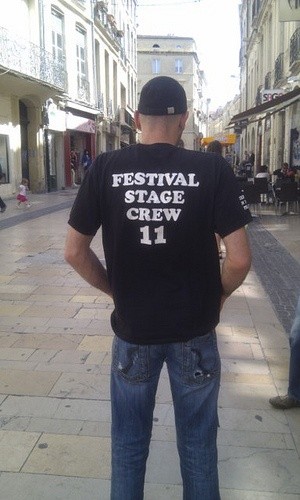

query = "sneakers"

[269,394,300,408]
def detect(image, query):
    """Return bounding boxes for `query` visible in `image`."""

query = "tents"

[201,133,236,146]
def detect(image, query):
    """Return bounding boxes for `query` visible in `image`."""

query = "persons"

[64,74,254,500]
[11,177,32,208]
[208,140,300,215]
[70,147,78,187]
[81,150,91,184]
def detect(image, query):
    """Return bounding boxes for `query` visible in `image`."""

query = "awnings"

[224,88,300,130]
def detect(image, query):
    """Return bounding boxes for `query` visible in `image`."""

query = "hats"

[138,76,187,114]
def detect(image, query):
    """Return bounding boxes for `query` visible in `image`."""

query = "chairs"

[236,176,300,216]
